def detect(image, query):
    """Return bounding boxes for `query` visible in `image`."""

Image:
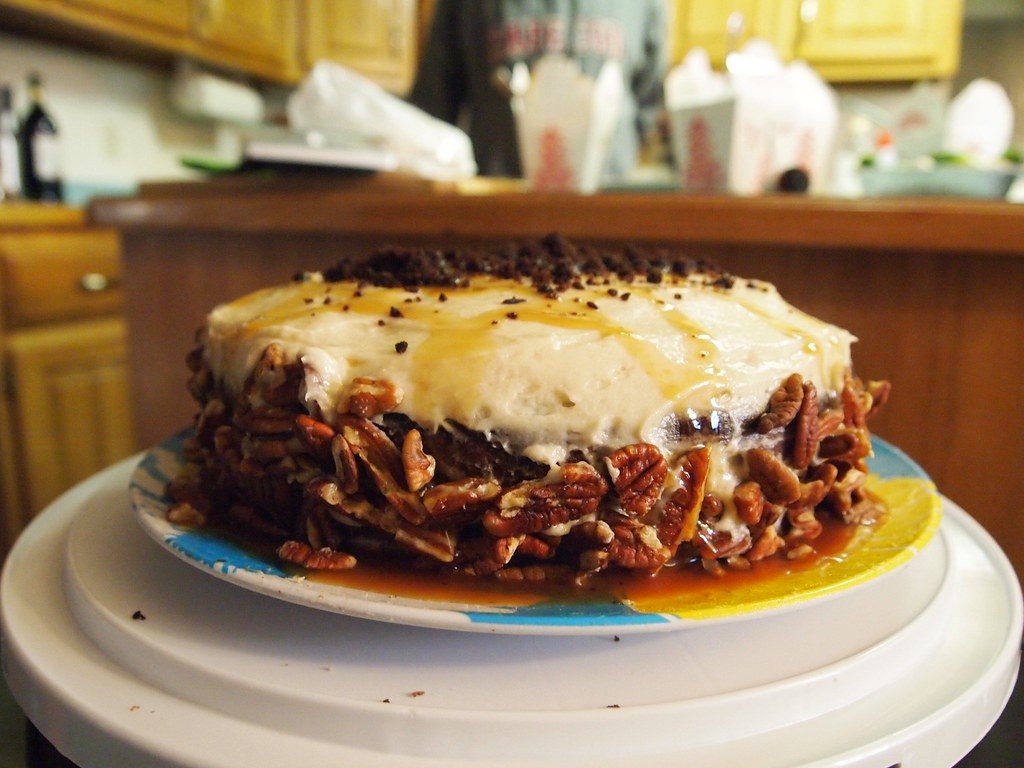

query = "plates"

[127,420,940,637]
[855,166,1016,202]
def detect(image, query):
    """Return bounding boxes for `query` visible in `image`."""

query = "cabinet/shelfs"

[0,0,419,97]
[0,200,136,566]
[80,181,1024,586]
[670,0,964,91]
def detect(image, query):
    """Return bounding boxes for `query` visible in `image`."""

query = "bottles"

[20,73,65,205]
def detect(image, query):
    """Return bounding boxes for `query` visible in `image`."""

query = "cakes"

[182,239,892,579]
